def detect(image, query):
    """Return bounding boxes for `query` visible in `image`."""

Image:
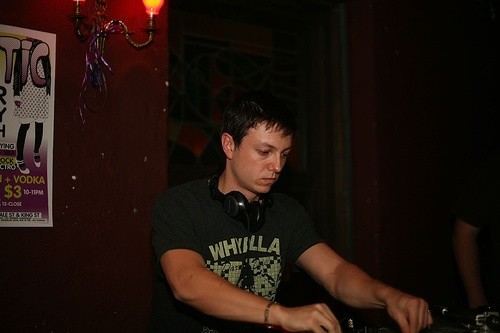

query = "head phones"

[208,172,274,233]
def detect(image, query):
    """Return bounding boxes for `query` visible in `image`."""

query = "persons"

[150,98,433,333]
[453,170,500,311]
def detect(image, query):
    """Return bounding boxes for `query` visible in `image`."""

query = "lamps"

[69,0,164,89]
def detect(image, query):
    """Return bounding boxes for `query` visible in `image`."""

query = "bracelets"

[264,301,276,329]
